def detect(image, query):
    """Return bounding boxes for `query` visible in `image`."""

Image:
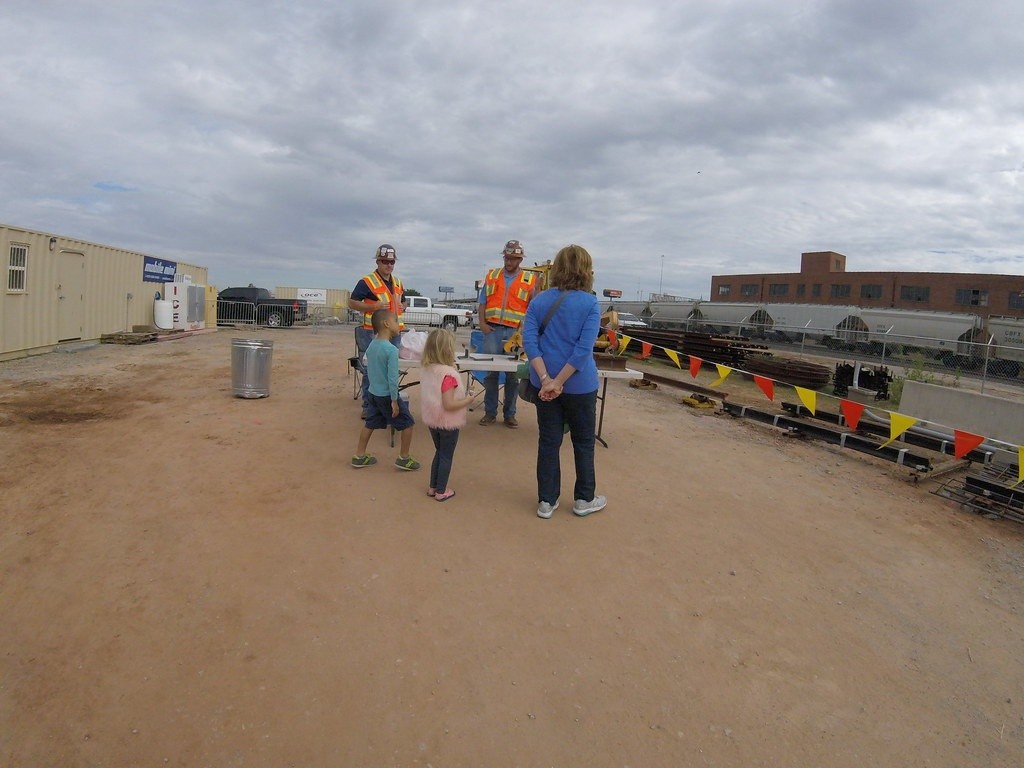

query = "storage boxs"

[593,352,629,372]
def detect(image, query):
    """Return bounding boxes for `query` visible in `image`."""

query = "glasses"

[503,256,517,261]
[381,260,396,265]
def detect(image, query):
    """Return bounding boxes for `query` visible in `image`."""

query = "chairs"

[465,331,507,412]
[347,325,408,400]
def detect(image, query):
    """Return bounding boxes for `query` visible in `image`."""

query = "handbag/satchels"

[518,378,539,404]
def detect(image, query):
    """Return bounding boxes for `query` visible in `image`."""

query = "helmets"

[372,243,399,261]
[499,240,527,257]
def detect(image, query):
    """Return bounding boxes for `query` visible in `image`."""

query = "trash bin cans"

[230,337,274,400]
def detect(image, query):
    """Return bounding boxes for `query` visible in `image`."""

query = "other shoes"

[504,417,519,429]
[427,486,437,497]
[435,488,455,502]
[361,404,368,419]
[480,413,496,426]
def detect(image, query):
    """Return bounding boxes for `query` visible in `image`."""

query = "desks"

[362,351,644,449]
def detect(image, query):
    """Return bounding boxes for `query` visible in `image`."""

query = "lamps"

[49,238,56,251]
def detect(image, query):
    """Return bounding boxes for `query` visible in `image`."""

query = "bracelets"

[540,372,548,382]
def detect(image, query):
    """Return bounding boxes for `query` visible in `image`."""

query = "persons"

[422,329,475,502]
[522,244,606,519]
[351,309,421,471]
[348,244,407,420]
[477,240,537,429]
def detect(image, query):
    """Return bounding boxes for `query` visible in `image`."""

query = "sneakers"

[573,495,607,516]
[351,453,377,468]
[394,455,421,471]
[537,499,560,518]
